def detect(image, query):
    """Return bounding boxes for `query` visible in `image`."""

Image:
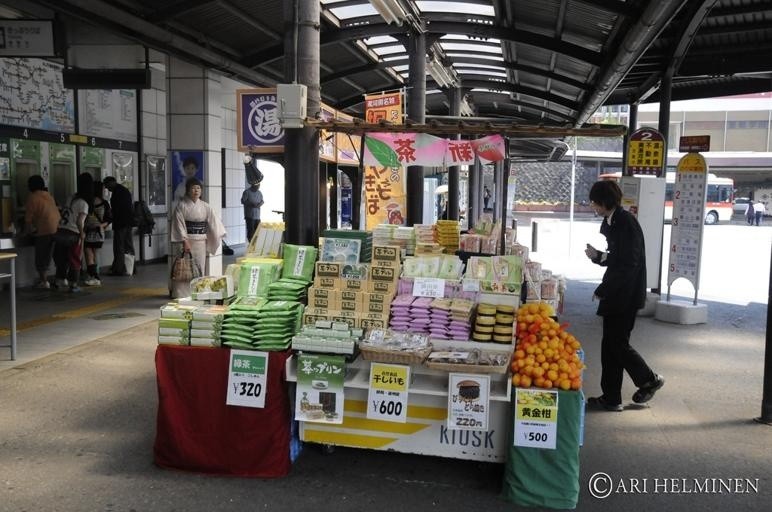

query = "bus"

[598,166,736,227]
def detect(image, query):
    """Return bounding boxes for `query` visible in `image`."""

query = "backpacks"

[133,200,155,234]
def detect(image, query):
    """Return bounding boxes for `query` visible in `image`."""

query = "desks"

[500,385,580,511]
[152,344,299,481]
[0,253,17,361]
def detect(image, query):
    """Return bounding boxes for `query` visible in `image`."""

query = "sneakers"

[32,275,102,293]
[631,373,665,403]
[587,394,623,411]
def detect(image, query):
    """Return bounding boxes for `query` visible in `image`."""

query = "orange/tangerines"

[510,300,583,391]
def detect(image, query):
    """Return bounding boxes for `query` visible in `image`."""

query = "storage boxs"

[321,229,372,263]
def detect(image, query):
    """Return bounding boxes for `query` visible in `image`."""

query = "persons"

[25,173,136,292]
[241,182,264,243]
[168,178,219,299]
[484,186,491,212]
[174,156,203,203]
[586,180,665,411]
[744,200,765,226]
[440,192,448,219]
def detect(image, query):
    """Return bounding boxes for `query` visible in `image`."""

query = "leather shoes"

[103,268,137,276]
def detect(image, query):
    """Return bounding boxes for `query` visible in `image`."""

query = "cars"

[731,196,754,215]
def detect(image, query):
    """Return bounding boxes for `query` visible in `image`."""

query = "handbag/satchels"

[70,245,81,272]
[170,257,204,283]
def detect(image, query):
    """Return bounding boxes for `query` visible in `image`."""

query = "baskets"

[359,342,433,365]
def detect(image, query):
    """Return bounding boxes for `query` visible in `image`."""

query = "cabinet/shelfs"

[288,266,524,493]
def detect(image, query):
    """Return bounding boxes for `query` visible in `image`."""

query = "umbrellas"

[433,185,448,204]
